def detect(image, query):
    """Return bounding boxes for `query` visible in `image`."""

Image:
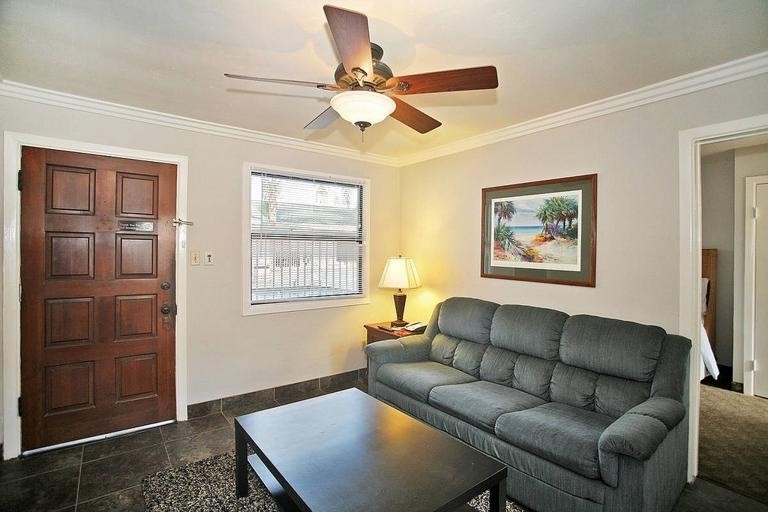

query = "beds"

[702,248,718,383]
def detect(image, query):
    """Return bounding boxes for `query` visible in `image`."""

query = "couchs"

[362,297,693,512]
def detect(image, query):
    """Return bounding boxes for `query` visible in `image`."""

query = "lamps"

[326,90,397,144]
[377,255,423,326]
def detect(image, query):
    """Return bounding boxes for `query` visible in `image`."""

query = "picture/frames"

[481,173,598,286]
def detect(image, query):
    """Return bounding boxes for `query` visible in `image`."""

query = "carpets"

[141,445,530,512]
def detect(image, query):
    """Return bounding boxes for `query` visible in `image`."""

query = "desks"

[364,320,426,388]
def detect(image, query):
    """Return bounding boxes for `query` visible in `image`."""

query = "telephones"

[405,321,428,332]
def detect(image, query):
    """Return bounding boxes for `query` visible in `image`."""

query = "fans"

[224,4,499,134]
